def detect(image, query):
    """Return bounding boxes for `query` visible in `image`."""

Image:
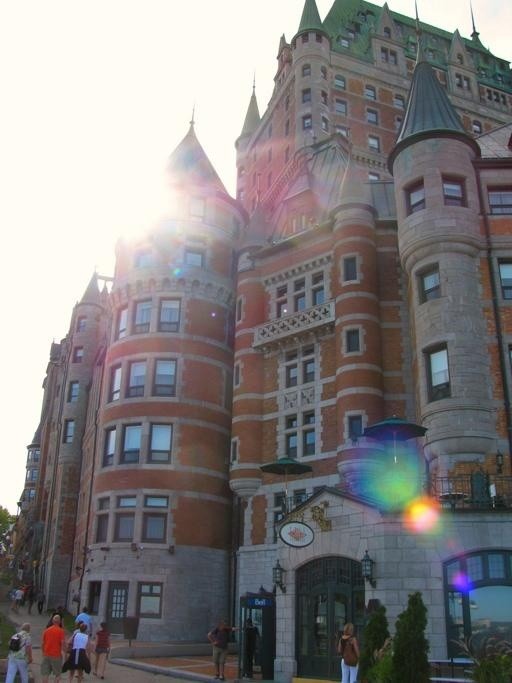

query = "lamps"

[495,448,505,474]
[360,550,377,588]
[272,558,287,593]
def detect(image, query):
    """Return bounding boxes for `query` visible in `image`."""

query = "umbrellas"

[363,412,427,461]
[261,453,312,493]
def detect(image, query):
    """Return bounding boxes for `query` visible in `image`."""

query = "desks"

[439,492,469,509]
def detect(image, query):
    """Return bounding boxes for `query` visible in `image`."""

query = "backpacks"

[10,633,26,652]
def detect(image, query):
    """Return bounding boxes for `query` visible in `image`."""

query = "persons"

[0,570,112,682]
[334,621,360,682]
[240,617,260,677]
[207,618,239,679]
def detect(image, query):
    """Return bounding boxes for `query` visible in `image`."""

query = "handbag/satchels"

[341,641,358,666]
